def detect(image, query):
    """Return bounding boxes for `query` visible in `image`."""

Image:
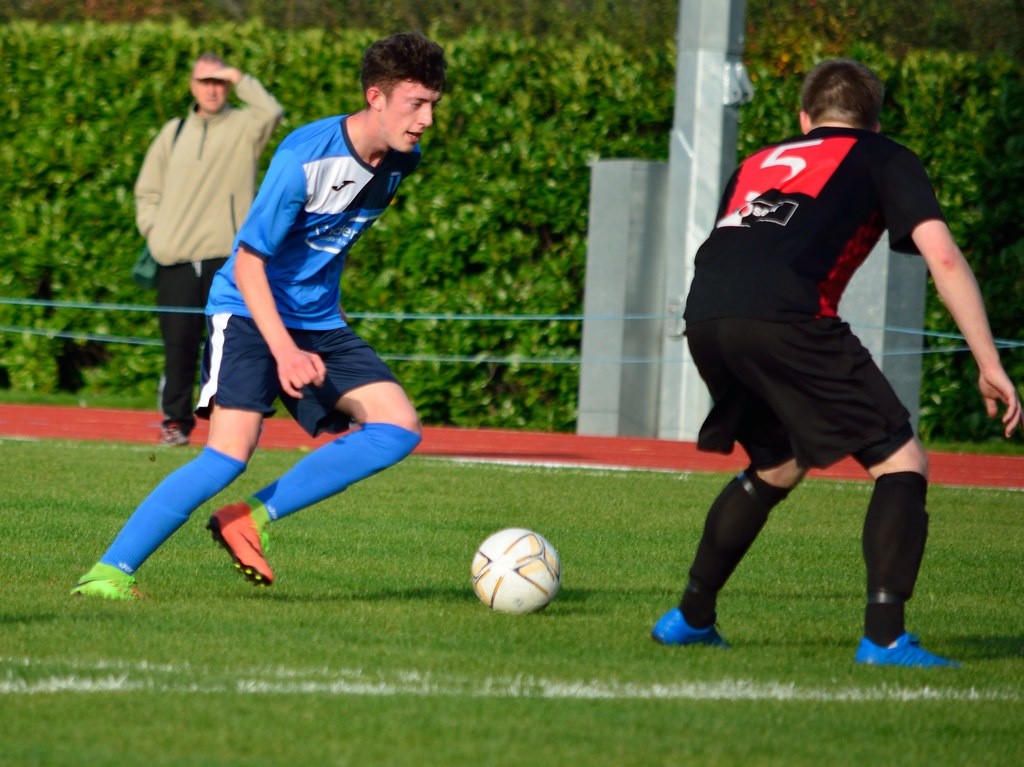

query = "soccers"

[470,527,560,615]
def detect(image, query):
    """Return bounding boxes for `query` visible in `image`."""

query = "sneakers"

[206,502,274,586]
[650,606,730,645]
[69,574,143,600]
[159,425,189,448]
[855,632,960,667]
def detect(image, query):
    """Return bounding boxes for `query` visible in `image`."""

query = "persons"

[649,57,1024,672]
[70,35,450,604]
[132,57,284,449]
